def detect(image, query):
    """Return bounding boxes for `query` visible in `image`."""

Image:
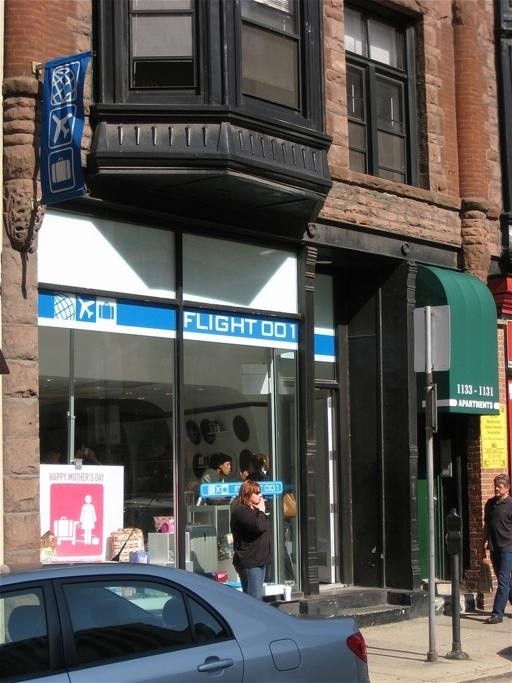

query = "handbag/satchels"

[283,493,296,518]
[111,527,145,563]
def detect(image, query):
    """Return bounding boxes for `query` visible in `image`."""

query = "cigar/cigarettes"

[260,498,267,501]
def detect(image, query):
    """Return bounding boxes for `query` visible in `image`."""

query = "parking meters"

[445,508,470,660]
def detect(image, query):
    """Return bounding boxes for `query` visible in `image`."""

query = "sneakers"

[487,615,503,624]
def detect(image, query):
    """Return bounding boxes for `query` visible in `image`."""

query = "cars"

[0,562,372,683]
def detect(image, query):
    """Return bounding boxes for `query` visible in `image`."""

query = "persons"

[245,453,269,481]
[230,480,273,602]
[43,437,102,464]
[198,452,250,505]
[477,472,511,624]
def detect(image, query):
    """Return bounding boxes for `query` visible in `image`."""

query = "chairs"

[8,603,44,641]
[163,598,187,628]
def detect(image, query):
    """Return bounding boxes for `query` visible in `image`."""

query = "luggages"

[185,491,219,575]
